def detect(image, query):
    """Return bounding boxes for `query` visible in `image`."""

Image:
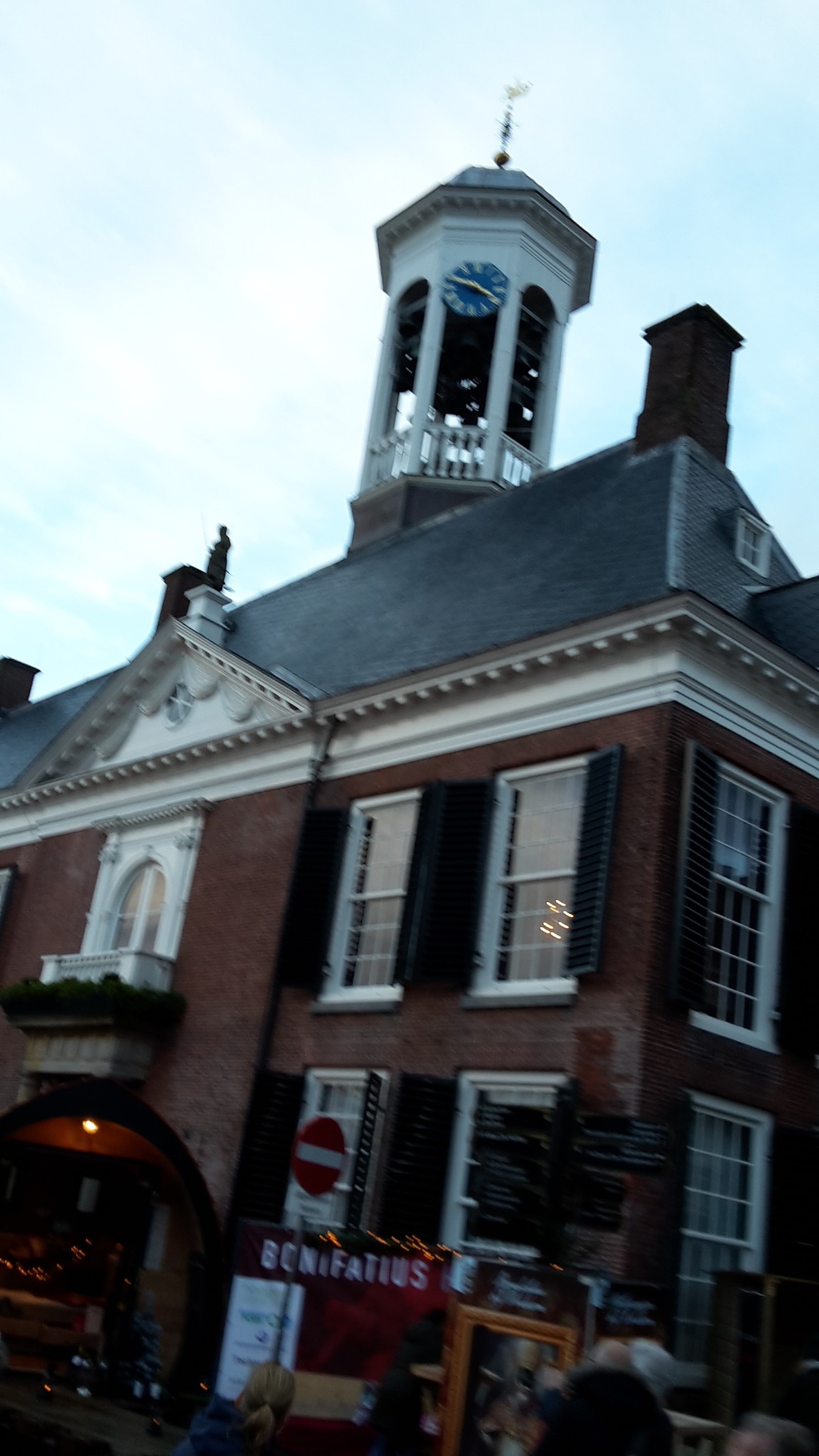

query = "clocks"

[441,260,509,317]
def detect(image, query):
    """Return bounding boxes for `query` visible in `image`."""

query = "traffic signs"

[471,1100,671,1249]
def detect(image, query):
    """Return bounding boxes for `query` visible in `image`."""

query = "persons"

[346,1343,444,1454]
[169,1361,299,1454]
[719,1410,817,1455]
[469,1336,680,1454]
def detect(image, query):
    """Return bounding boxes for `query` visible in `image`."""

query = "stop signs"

[293,1116,350,1198]
